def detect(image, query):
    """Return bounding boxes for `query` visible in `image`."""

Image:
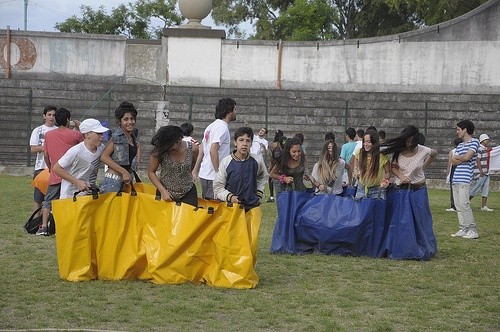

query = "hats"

[453,138,462,145]
[79,118,110,135]
[479,134,490,142]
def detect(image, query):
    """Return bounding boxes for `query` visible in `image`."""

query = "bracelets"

[278,174,286,184]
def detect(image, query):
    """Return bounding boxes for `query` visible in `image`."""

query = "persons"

[179,122,201,151]
[37,108,85,236]
[352,130,391,199]
[312,140,354,197]
[263,129,285,203]
[53,118,110,198]
[270,137,311,193]
[379,124,438,186]
[191,97,238,199]
[148,125,200,209]
[252,128,268,161]
[293,124,426,185]
[446,133,493,212]
[99,100,142,194]
[450,120,480,239]
[29,105,58,219]
[212,127,267,211]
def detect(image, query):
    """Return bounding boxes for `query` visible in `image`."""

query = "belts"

[104,171,123,182]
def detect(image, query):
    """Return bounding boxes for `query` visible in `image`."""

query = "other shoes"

[481,206,493,212]
[463,230,479,239]
[451,230,467,237]
[265,197,274,203]
[445,208,455,212]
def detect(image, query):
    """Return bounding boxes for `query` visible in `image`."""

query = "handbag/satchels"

[23,206,55,234]
